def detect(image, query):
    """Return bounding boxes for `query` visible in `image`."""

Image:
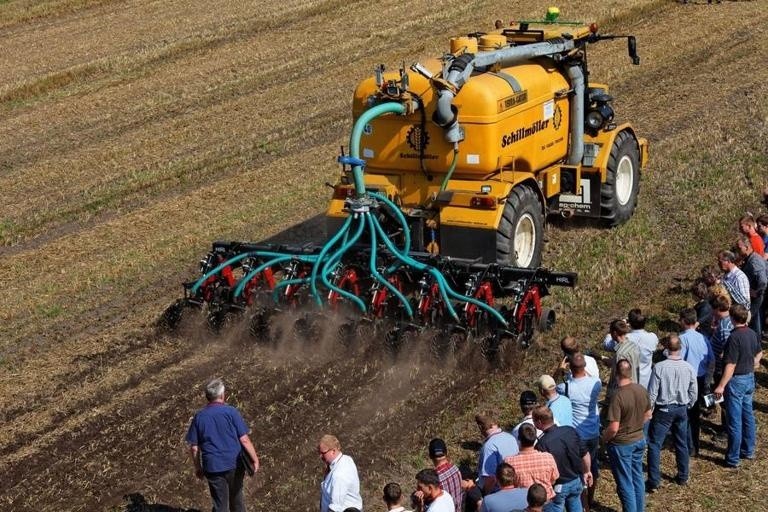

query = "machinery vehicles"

[156,22,644,371]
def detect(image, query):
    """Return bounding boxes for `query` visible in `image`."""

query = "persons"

[318,434,363,511]
[184,379,259,511]
[382,213,767,510]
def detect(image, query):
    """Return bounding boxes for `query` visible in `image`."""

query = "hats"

[429,438,446,451]
[520,390,537,405]
[537,374,556,390]
